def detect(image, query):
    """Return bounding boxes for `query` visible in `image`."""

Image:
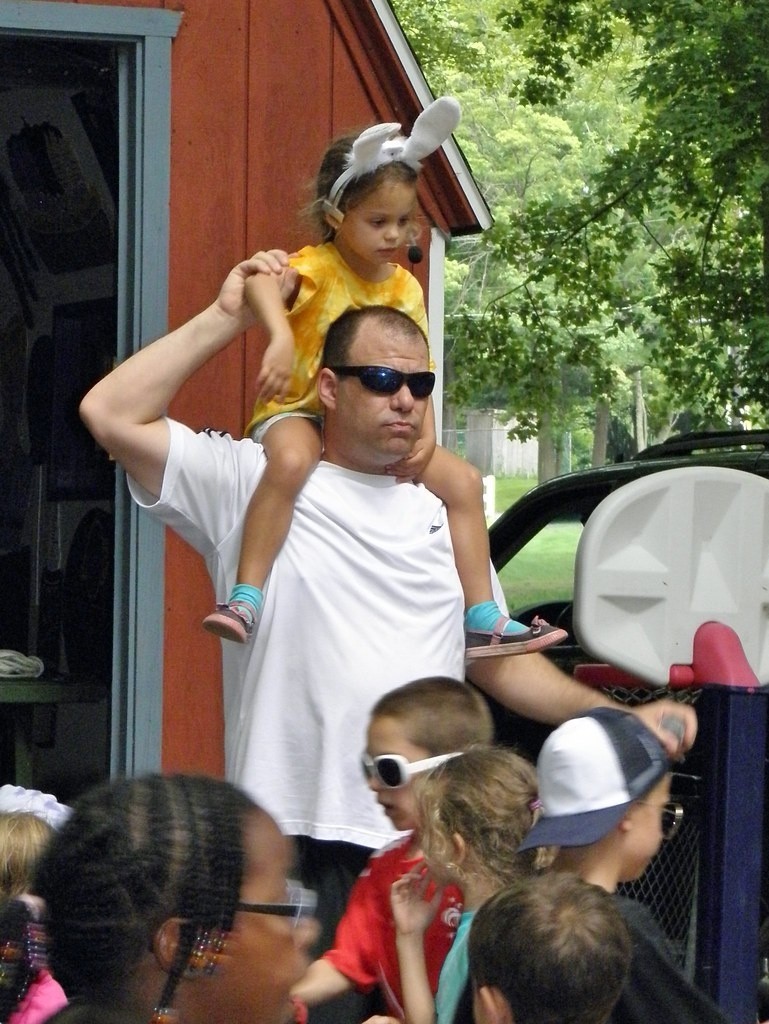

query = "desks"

[0,680,100,789]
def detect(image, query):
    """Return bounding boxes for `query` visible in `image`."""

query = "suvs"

[484,427,769,995]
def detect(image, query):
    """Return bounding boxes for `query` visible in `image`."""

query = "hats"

[513,707,669,855]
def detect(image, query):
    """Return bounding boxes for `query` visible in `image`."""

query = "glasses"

[633,800,684,839]
[149,876,318,955]
[364,751,465,792]
[333,366,435,398]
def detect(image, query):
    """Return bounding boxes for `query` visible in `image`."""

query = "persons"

[0,784,75,904]
[290,677,493,1024]
[469,870,636,1024]
[79,251,698,1024]
[201,95,569,661]
[518,706,735,1024]
[392,745,561,1023]
[0,772,400,1024]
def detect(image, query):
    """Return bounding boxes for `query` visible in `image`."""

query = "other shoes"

[201,602,253,645]
[466,616,568,659]
